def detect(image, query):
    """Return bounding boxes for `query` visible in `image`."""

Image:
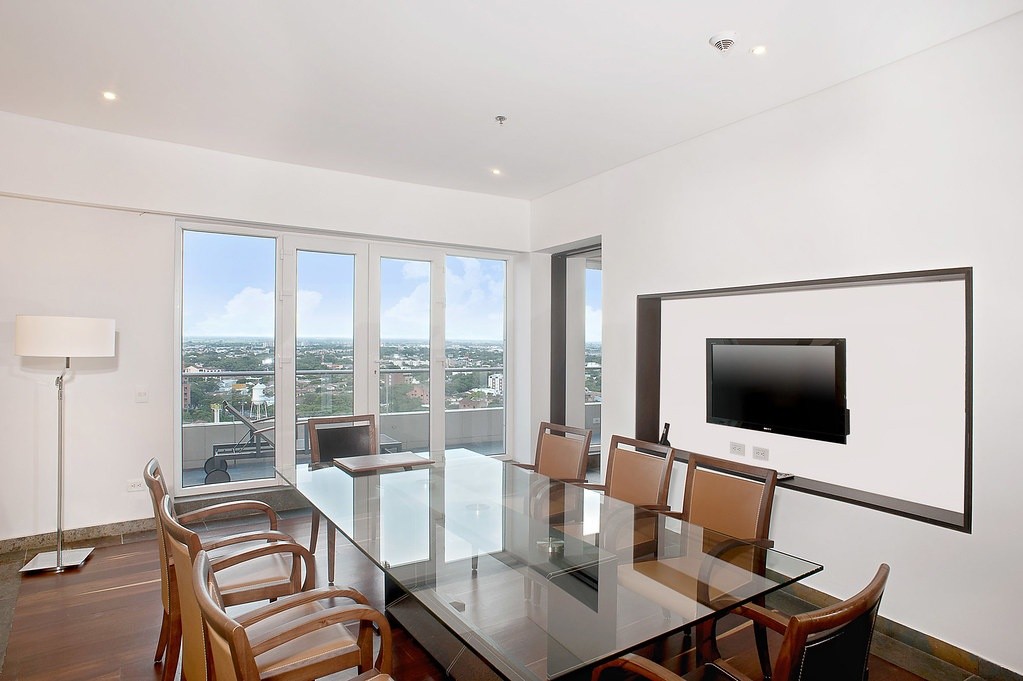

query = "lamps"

[16,314,114,572]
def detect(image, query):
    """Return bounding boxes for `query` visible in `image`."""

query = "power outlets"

[126,479,145,491]
[752,446,769,460]
[730,441,745,456]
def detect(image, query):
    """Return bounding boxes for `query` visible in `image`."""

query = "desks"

[274,447,823,681]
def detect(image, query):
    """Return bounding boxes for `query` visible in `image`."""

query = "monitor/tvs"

[706,338,850,444]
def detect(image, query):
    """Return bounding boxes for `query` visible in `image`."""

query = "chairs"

[143,458,315,681]
[590,563,890,681]
[470,423,592,598]
[190,550,394,681]
[205,401,413,485]
[308,413,378,582]
[613,453,778,679]
[525,435,676,621]
[160,494,375,680]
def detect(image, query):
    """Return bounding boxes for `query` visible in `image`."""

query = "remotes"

[777,473,794,479]
